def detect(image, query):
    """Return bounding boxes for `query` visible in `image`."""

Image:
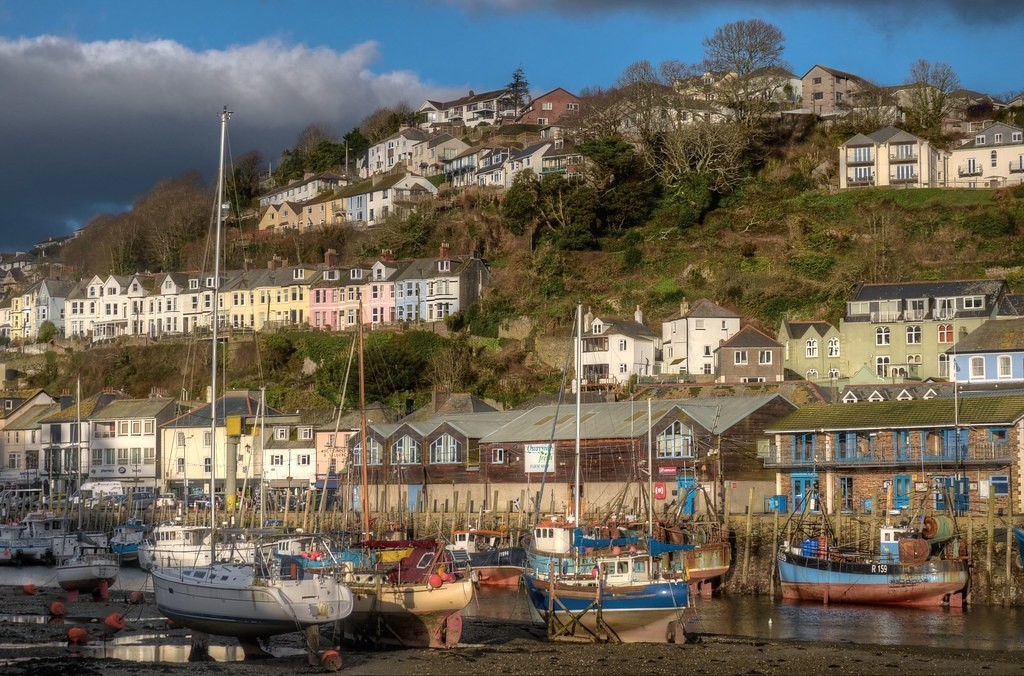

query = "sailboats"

[0,104,1024,646]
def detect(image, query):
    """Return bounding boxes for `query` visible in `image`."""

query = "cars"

[99,491,342,512]
[0,488,70,511]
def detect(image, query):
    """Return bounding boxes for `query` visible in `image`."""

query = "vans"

[67,480,124,509]
